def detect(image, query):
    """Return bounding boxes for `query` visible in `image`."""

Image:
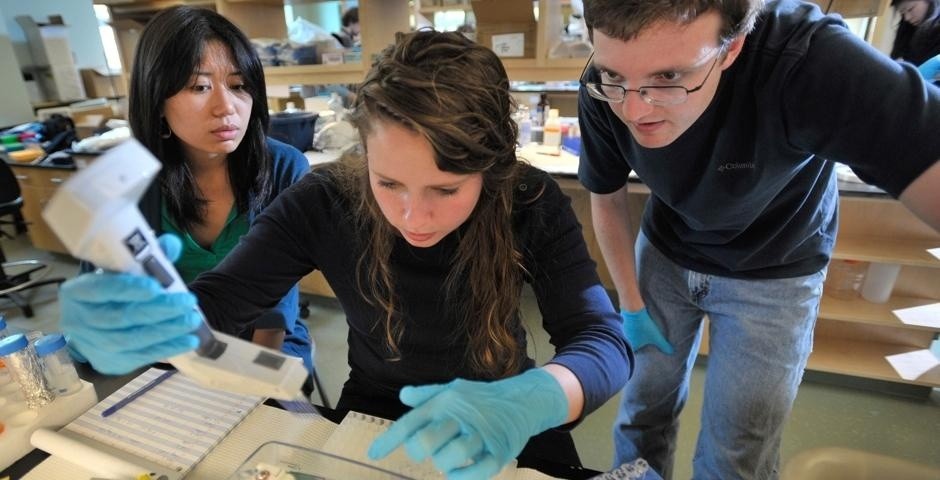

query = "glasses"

[579,45,725,105]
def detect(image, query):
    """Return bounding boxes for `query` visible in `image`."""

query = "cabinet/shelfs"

[10,166,87,259]
[109,0,561,86]
[585,169,932,401]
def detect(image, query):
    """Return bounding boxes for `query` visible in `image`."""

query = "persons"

[580,0,940,480]
[56,25,635,480]
[890,0,939,67]
[335,7,359,47]
[66,4,314,398]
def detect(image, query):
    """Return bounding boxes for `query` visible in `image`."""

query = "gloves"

[620,308,676,357]
[368,367,569,480]
[60,234,202,378]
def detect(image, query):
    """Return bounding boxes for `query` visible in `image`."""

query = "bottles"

[0,334,54,410]
[520,107,532,145]
[830,259,864,302]
[36,331,81,395]
[328,92,341,121]
[543,108,560,147]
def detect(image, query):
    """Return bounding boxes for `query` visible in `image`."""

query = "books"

[30,368,268,480]
[325,410,560,480]
[589,457,663,480]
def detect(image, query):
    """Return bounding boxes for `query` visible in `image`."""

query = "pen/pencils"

[101,368,177,418]
